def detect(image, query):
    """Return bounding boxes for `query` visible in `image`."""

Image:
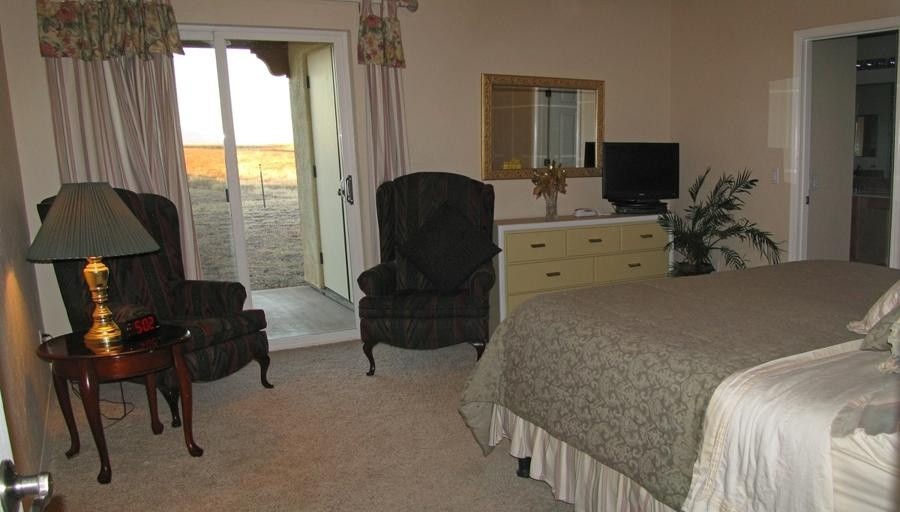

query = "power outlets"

[38,330,44,345]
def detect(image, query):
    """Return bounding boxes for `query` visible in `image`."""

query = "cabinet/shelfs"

[489,213,675,342]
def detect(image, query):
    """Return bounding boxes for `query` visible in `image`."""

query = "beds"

[457,258,900,512]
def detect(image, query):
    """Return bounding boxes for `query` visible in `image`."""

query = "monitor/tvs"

[601,142,680,207]
[584,141,595,167]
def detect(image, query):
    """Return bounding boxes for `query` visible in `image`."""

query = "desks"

[37,331,203,484]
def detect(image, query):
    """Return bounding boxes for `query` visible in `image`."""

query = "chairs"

[37,187,274,427]
[357,172,496,376]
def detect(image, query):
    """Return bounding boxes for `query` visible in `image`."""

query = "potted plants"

[657,165,790,276]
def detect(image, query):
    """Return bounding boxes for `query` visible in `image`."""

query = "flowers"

[531,158,568,200]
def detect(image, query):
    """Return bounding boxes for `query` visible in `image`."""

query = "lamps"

[25,181,161,341]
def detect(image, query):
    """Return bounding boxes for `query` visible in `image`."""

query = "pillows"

[861,308,900,352]
[845,275,900,335]
[398,198,501,295]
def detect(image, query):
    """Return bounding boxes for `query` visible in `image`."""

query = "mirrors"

[853,113,880,157]
[479,71,606,181]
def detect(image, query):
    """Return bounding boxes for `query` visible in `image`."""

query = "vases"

[546,196,558,221]
